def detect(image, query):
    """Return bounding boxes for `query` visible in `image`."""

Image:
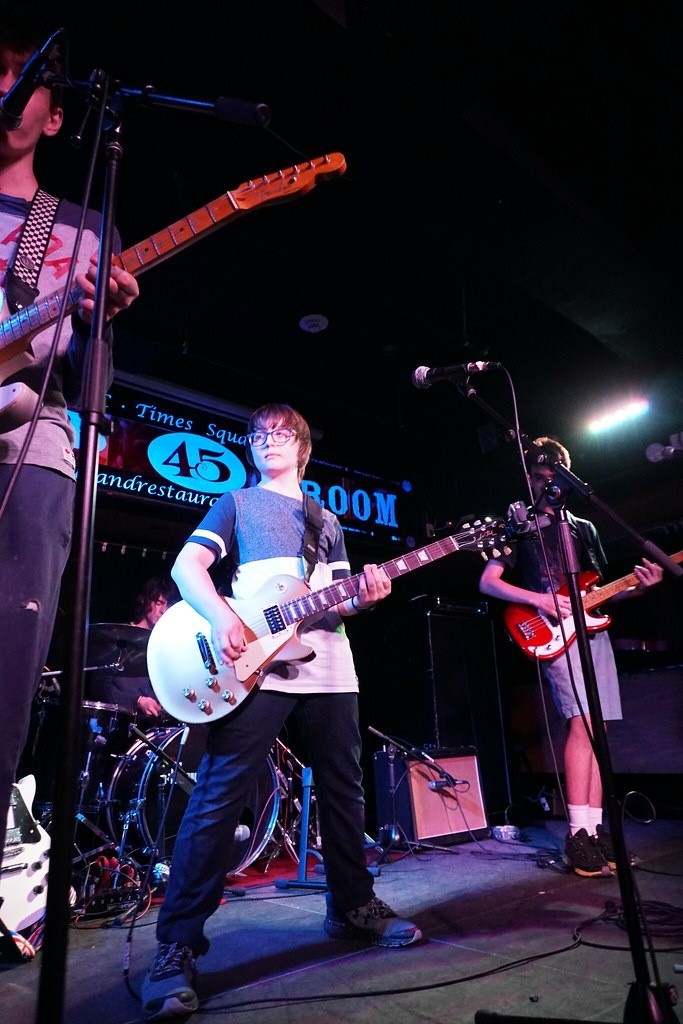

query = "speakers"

[415,610,512,816]
[372,745,492,850]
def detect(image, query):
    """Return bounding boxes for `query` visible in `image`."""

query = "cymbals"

[58,614,151,678]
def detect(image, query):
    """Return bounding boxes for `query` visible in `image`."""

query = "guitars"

[0,774,78,935]
[503,551,683,664]
[148,517,515,729]
[1,149,348,441]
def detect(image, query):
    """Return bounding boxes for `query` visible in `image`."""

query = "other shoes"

[152,863,169,884]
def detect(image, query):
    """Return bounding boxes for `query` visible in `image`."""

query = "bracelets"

[635,587,644,593]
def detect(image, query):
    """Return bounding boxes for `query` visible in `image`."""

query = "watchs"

[352,595,375,614]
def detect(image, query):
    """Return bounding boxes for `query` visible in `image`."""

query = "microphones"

[234,823,250,842]
[413,361,500,388]
[0,28,67,131]
[428,781,466,790]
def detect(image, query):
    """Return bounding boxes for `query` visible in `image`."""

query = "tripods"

[361,726,464,868]
[263,727,384,876]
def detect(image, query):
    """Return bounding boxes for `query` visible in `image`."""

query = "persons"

[140,404,424,1018]
[101,576,182,884]
[479,437,664,879]
[0,30,139,867]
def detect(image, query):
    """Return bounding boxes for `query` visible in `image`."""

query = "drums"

[107,728,281,879]
[73,700,137,815]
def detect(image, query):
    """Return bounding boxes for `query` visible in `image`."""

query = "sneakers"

[323,892,422,948]
[590,824,640,868]
[140,941,199,1019]
[561,827,614,877]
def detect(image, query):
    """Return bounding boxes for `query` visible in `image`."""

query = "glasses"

[249,428,296,447]
